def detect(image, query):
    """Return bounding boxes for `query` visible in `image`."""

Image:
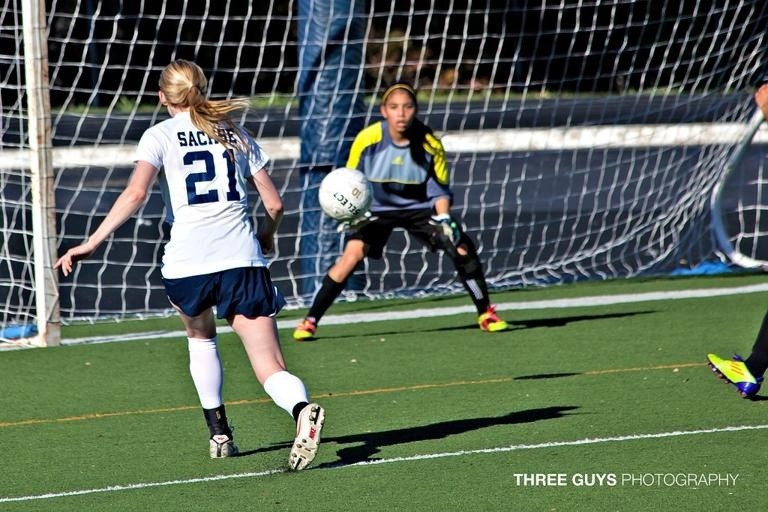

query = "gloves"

[337,211,379,236]
[428,214,462,249]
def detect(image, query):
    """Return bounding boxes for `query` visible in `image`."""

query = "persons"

[58,58,326,473]
[290,80,510,340]
[704,77,766,401]
[292,22,374,308]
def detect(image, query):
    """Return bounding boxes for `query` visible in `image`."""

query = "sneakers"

[478,304,509,332]
[294,317,316,341]
[705,352,764,399]
[288,403,326,471]
[209,434,238,458]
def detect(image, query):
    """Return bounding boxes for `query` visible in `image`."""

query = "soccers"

[318,166,374,222]
